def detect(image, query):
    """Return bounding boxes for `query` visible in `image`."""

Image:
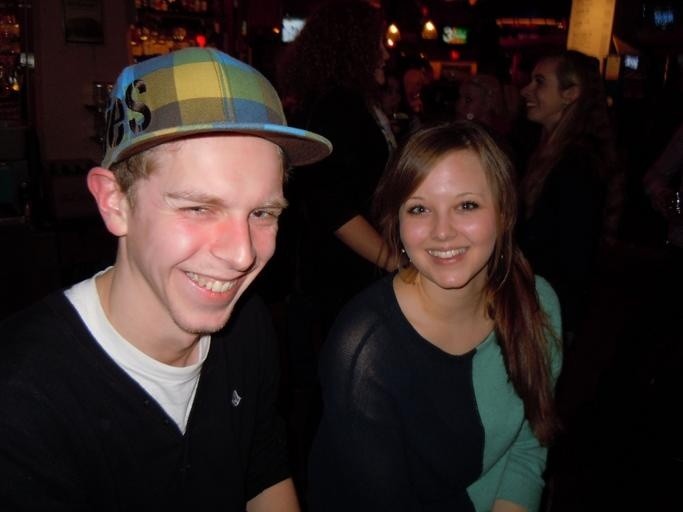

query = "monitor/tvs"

[280,14,307,44]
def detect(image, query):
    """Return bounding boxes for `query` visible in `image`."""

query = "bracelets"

[376,237,391,271]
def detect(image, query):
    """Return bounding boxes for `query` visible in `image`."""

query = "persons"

[285,2,407,312]
[515,49,626,280]
[325,119,562,511]
[0,47,336,512]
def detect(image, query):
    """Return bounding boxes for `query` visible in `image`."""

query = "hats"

[101,47,333,169]
